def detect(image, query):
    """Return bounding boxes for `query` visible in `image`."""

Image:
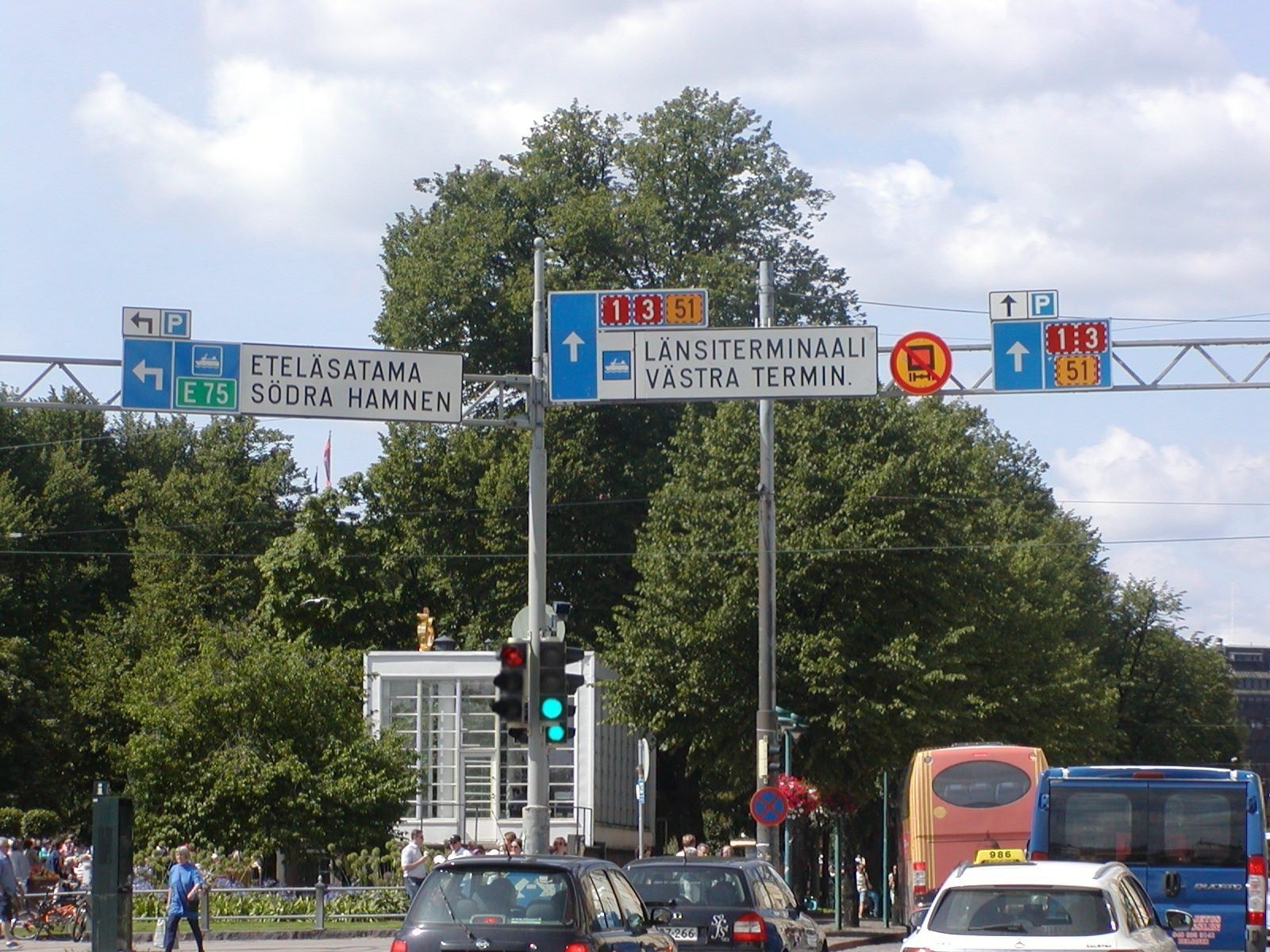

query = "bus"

[1024,766,1270,952]
[898,741,1049,940]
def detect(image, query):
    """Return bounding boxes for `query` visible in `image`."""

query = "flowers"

[776,773,821,820]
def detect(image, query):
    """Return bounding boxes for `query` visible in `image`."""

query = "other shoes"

[5,941,23,950]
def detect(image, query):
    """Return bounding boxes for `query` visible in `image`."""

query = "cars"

[509,793,526,816]
[548,790,574,818]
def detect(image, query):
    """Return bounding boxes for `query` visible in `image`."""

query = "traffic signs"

[124,339,463,424]
[549,290,880,402]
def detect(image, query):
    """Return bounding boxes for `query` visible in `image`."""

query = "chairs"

[470,884,508,916]
[970,784,995,803]
[706,881,740,906]
[946,788,972,807]
[966,894,1017,932]
[998,785,1025,804]
[524,898,561,921]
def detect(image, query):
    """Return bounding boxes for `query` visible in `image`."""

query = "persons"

[635,843,651,884]
[490,878,517,914]
[447,835,472,898]
[856,864,867,919]
[676,835,734,904]
[888,864,896,905]
[164,846,204,952]
[0,837,22,950]
[401,830,427,906]
[500,832,523,854]
[132,856,154,897]
[550,837,567,893]
[11,834,93,914]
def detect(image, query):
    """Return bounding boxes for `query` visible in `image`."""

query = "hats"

[448,835,462,842]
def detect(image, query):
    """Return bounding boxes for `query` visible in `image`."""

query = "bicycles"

[9,884,89,942]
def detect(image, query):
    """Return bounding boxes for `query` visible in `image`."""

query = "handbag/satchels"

[153,917,179,949]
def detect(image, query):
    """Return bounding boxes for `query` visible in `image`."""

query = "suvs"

[389,811,679,952]
[901,848,1194,952]
[620,847,830,952]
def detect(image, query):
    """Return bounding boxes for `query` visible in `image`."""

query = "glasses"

[560,842,568,847]
[510,845,518,848]
[416,835,423,839]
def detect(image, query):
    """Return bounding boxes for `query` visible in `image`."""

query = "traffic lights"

[762,736,782,782]
[490,639,531,725]
[540,641,584,744]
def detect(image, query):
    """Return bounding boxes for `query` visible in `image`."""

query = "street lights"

[773,706,813,894]
[880,765,899,929]
